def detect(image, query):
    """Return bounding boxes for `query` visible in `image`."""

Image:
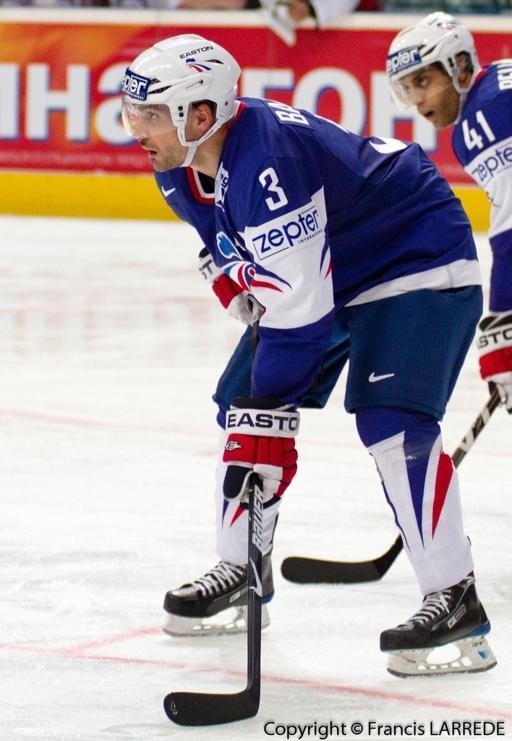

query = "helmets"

[386,11,482,112]
[121,33,242,139]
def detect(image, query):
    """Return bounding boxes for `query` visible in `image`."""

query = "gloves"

[197,251,261,326]
[221,407,299,514]
[478,312,512,417]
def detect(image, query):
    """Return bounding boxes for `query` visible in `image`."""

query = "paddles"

[164,297,266,725]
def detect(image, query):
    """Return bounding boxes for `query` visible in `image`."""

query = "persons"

[382,13,512,416]
[115,32,494,651]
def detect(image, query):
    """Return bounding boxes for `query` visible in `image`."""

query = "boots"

[381,537,493,653]
[162,512,281,616]
